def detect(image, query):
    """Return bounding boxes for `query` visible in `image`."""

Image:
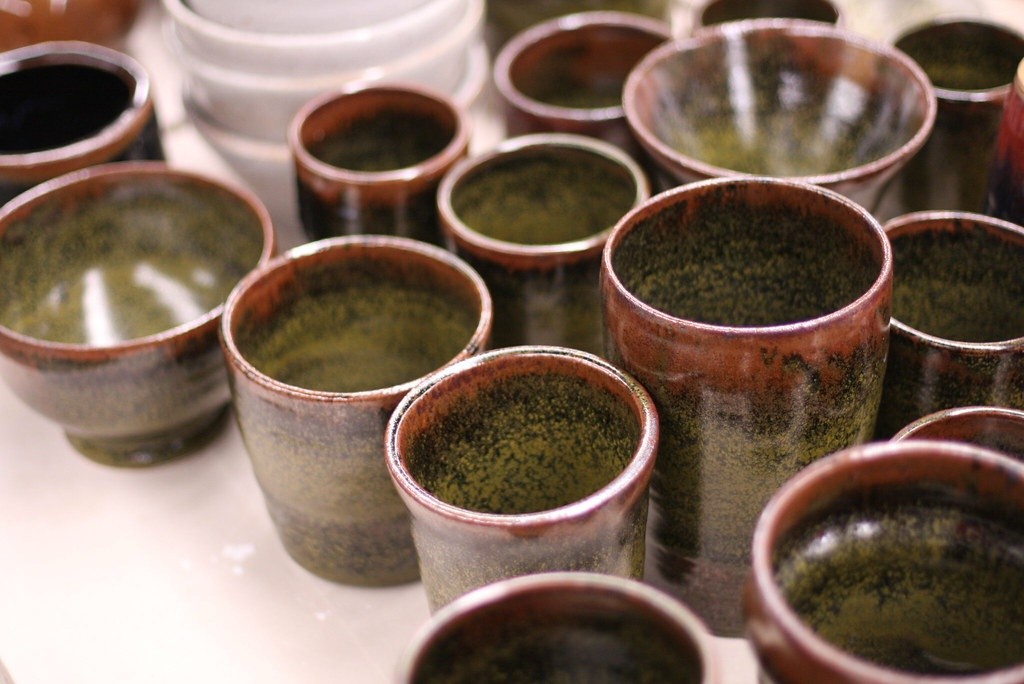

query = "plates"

[0,0,1023,683]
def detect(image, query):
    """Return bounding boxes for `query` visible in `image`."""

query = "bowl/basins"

[161,0,487,224]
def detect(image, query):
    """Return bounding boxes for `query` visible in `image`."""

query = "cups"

[494,10,672,192]
[880,211,1024,438]
[896,21,1024,216]
[393,575,721,684]
[386,346,656,615]
[288,81,467,242]
[694,0,843,32]
[437,133,648,354]
[623,19,935,215]
[745,444,1024,684]
[0,42,164,200]
[0,161,275,467]
[891,405,1024,462]
[222,235,491,588]
[601,176,892,638]
